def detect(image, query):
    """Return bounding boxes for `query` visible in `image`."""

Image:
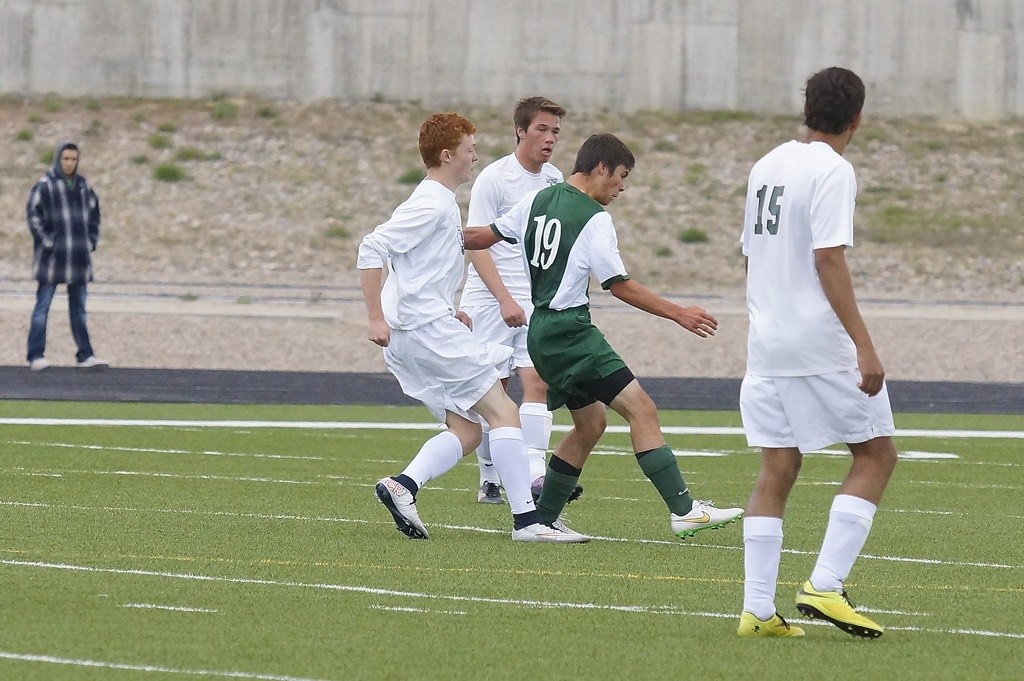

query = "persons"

[736,67,898,639]
[462,133,743,540]
[25,141,109,374]
[461,95,584,506]
[355,111,590,543]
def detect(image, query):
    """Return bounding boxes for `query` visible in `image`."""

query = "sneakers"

[670,499,745,540]
[478,481,507,504]
[30,357,51,372]
[795,580,884,640]
[511,523,585,543]
[374,477,429,540]
[76,356,109,369]
[736,611,806,639]
[530,475,583,507]
[551,517,592,542]
